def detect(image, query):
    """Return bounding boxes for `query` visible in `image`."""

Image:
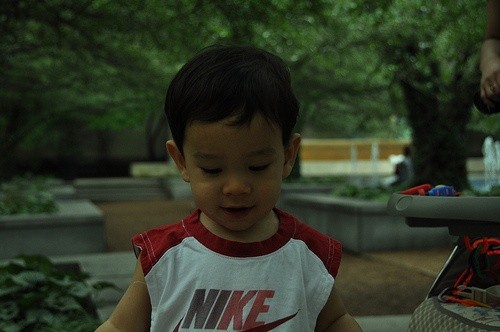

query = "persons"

[92,45,366,332]
[389,144,416,193]
